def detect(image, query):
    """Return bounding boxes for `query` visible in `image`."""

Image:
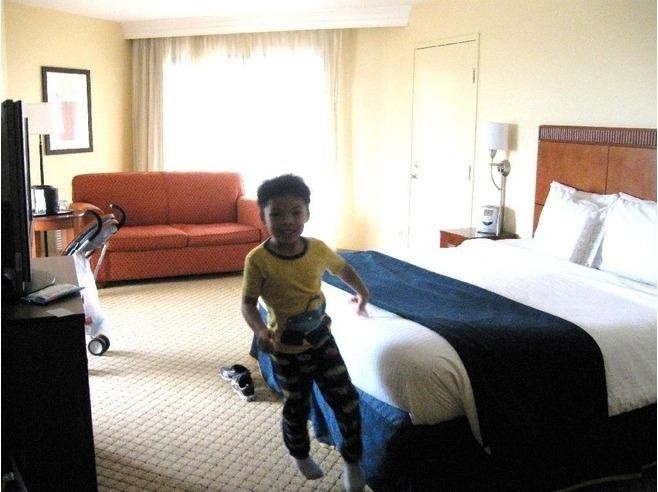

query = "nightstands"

[440,227,517,248]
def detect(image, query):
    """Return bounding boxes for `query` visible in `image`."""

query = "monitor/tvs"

[1,98,56,297]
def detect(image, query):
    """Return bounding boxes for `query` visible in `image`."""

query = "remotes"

[34,284,82,304]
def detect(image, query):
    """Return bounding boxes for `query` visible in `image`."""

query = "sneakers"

[220,364,251,381]
[231,374,257,401]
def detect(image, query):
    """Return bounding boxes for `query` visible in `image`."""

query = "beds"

[249,125,656,491]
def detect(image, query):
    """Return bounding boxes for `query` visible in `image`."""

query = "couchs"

[61,172,270,288]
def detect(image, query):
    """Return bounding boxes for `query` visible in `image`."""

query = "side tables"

[33,214,74,258]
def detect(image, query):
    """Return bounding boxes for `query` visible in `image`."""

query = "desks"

[1,256,99,492]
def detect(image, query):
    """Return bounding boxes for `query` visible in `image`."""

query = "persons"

[241,173,369,491]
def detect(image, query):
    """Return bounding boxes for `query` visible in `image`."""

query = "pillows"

[534,181,656,298]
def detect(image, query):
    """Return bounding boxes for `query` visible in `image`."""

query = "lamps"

[22,102,65,217]
[476,124,519,238]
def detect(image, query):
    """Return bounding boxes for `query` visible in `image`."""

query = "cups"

[58,199,68,212]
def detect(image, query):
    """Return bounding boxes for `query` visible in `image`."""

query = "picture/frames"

[41,66,94,156]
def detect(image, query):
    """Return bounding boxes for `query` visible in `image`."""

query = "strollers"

[60,203,126,354]
